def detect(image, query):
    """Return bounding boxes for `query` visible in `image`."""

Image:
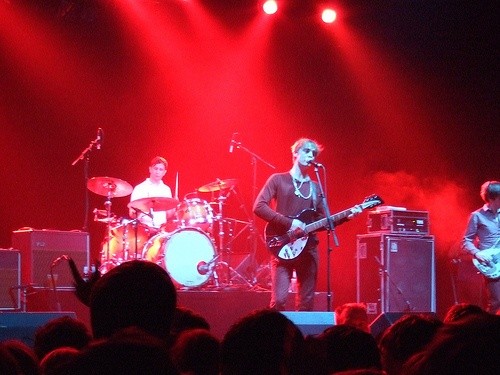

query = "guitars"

[472,240,500,280]
[265,193,384,261]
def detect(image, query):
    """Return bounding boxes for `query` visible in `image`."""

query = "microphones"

[309,160,323,168]
[229,134,235,153]
[199,265,209,272]
[226,186,234,197]
[96,129,101,149]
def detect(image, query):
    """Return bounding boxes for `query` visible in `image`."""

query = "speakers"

[383,237,435,313]
[0,312,77,350]
[369,313,404,348]
[12,231,89,289]
[0,248,21,312]
[280,311,336,336]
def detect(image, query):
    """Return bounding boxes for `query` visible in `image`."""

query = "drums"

[100,219,149,270]
[142,227,217,288]
[175,198,214,230]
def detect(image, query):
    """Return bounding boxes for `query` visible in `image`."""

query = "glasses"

[303,148,317,157]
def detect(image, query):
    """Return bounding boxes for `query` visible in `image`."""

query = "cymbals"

[126,197,179,212]
[87,176,133,197]
[199,179,239,192]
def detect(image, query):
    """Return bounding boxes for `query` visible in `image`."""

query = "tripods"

[210,186,254,291]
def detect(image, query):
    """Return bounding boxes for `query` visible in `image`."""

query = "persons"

[0,258,500,375]
[461,181,500,315]
[253,138,363,311]
[128,156,179,231]
